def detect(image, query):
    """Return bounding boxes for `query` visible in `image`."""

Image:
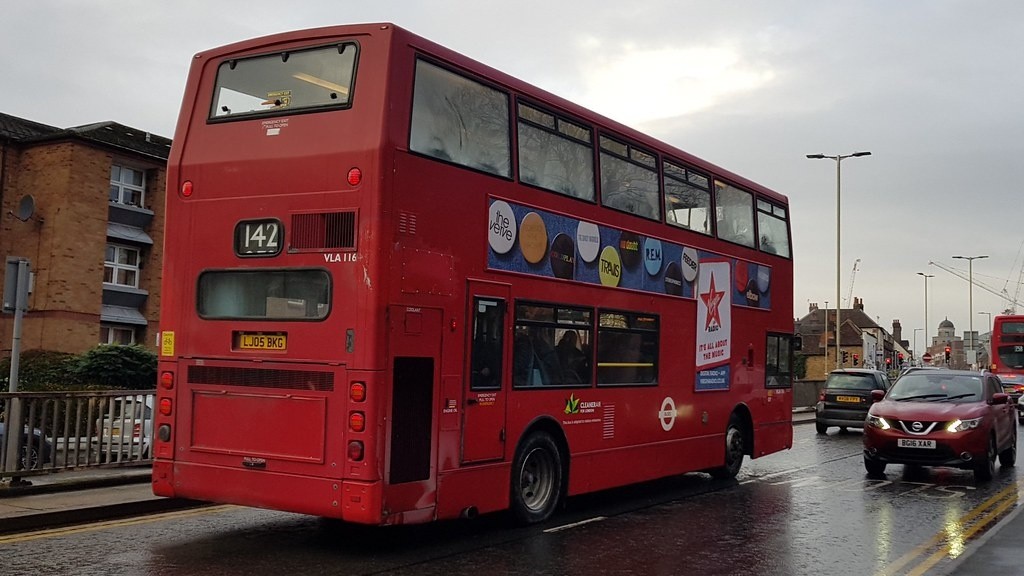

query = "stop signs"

[922,352,932,363]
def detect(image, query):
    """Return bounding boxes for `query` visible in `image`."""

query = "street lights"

[806,147,871,364]
[951,254,989,349]
[912,327,925,365]
[977,309,991,336]
[916,272,936,351]
[876,315,880,323]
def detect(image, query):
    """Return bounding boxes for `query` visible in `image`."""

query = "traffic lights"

[897,353,903,366]
[853,354,859,366]
[945,348,950,363]
[843,351,848,364]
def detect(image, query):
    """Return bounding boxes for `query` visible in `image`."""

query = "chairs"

[511,328,580,386]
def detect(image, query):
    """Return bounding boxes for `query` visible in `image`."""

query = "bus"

[146,19,804,534]
[988,314,1024,382]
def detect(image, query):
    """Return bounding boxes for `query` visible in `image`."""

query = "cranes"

[846,258,860,308]
[927,239,1024,309]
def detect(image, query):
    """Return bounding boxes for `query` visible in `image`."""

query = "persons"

[554,329,587,384]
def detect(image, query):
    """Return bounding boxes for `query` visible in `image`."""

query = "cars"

[862,367,1017,482]
[996,373,1024,401]
[813,367,892,435]
[0,420,54,468]
[886,371,897,380]
[900,361,935,375]
[1017,395,1024,425]
[95,395,153,461]
